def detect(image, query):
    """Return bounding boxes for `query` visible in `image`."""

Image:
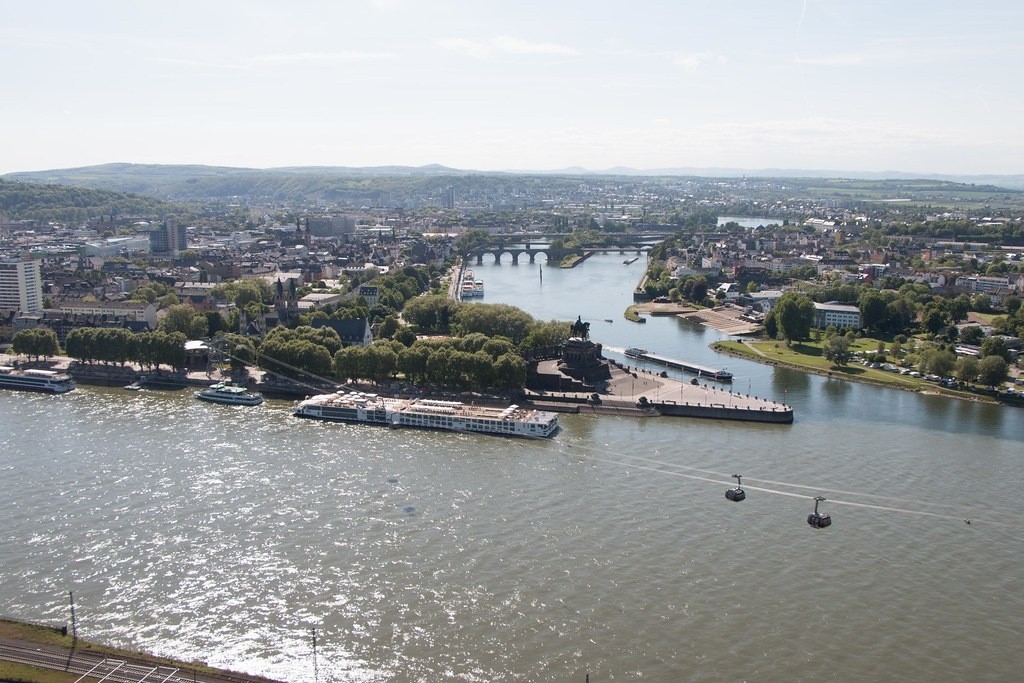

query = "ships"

[0,366,77,394]
[294,391,559,438]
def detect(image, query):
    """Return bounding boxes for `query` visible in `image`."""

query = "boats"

[623,345,733,382]
[461,267,484,298]
[623,256,639,265]
[193,380,264,406]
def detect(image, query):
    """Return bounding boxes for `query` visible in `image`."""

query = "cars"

[837,348,1024,400]
